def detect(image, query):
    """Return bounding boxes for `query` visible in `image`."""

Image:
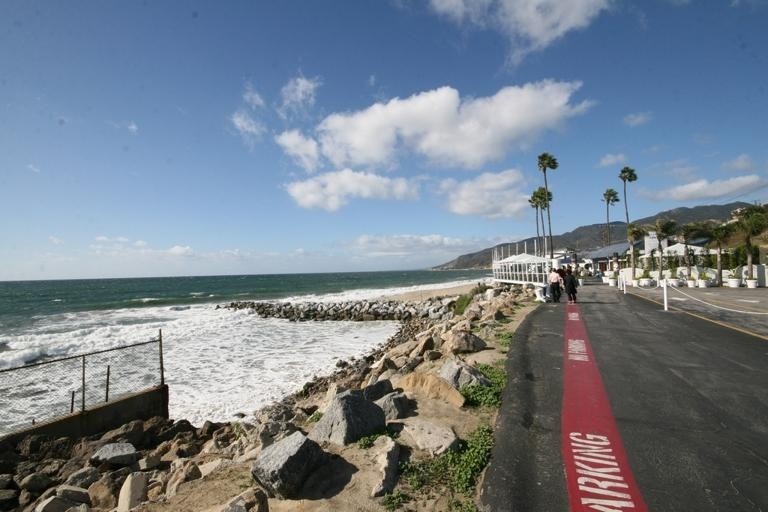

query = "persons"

[564,269,577,304]
[548,267,564,303]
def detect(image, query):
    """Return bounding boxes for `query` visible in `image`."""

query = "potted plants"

[607,263,761,289]
[578,276,584,287]
